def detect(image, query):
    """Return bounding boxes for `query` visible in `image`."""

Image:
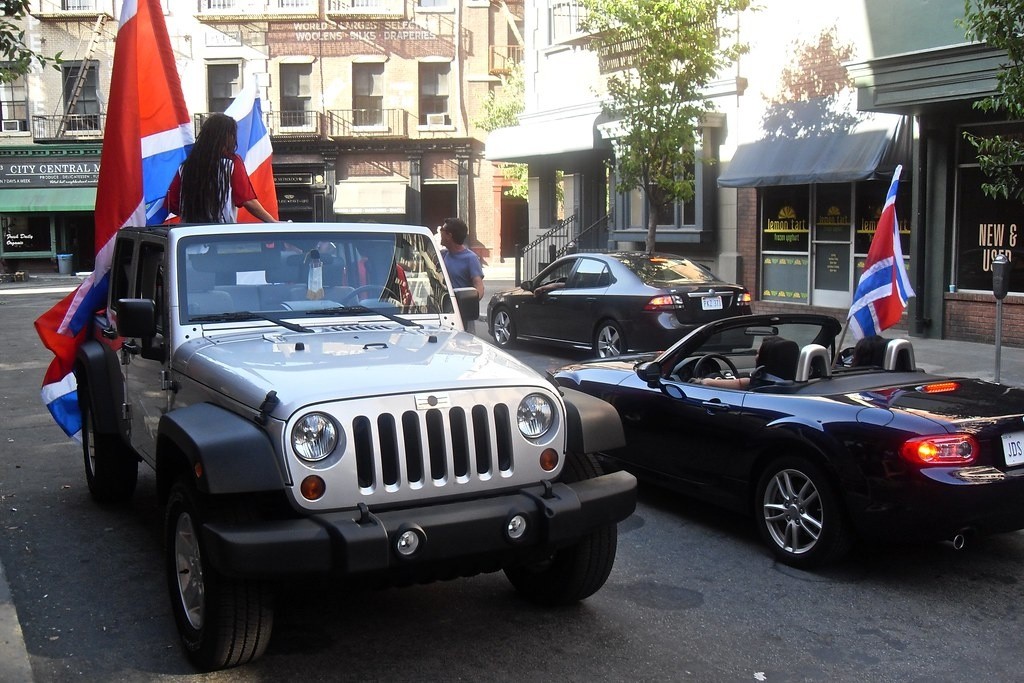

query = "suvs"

[77,224,641,672]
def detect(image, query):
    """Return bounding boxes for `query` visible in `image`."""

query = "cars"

[486,251,754,359]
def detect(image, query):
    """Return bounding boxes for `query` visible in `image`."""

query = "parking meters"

[993,254,1011,383]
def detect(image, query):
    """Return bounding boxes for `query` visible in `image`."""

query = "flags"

[846,165,917,341]
[34,0,277,438]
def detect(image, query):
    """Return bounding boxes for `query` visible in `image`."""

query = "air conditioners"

[1,120,19,131]
[427,114,445,125]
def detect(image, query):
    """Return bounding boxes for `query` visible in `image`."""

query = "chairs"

[187,256,358,318]
[750,335,886,387]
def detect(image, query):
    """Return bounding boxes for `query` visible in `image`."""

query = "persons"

[160,113,277,224]
[439,218,485,335]
[688,336,783,390]
[533,282,565,298]
[341,253,415,306]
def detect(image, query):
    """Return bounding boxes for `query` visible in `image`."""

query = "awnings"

[0,187,97,212]
[333,182,407,215]
[715,90,914,188]
[483,113,625,163]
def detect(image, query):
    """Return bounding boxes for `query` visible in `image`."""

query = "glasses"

[440,226,447,233]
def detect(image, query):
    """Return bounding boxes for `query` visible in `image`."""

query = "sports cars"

[544,313,1024,573]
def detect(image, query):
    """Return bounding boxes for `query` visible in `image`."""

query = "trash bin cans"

[56,254,73,273]
